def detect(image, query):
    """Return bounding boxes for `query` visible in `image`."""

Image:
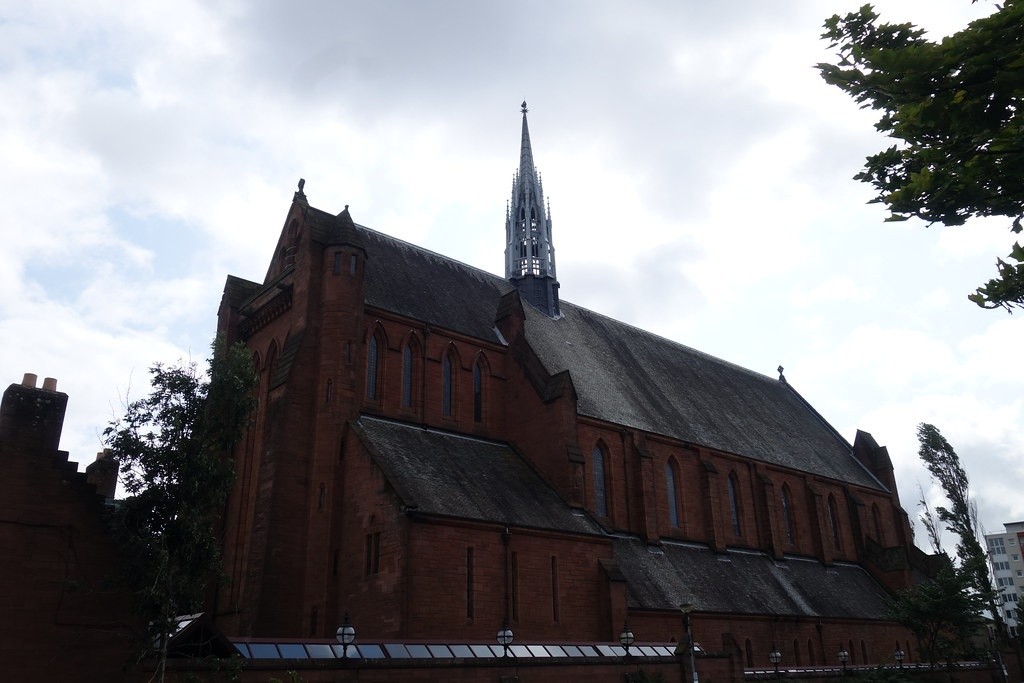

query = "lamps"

[769,643,783,673]
[496,616,515,657]
[620,620,634,656]
[837,643,849,671]
[894,646,905,668]
[335,610,356,658]
